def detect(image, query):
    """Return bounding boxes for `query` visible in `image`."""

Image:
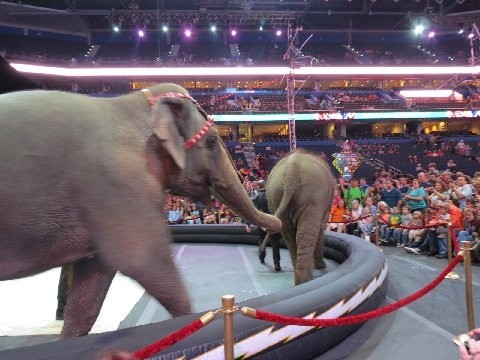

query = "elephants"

[258,149,337,285]
[0,82,282,342]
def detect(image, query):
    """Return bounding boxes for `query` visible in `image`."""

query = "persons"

[230,145,284,193]
[331,161,480,262]
[465,97,473,110]
[329,92,381,110]
[459,328,480,359]
[316,130,480,264]
[164,193,236,224]
[409,102,415,108]
[204,96,261,111]
